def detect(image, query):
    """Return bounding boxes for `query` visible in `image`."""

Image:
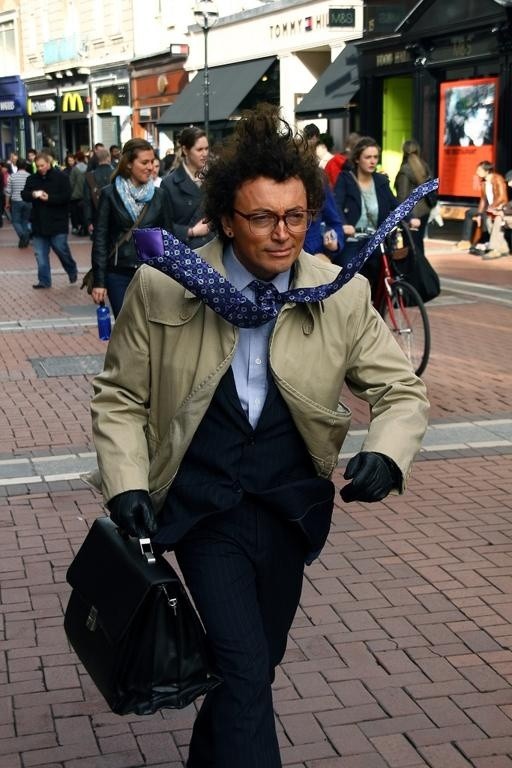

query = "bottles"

[96,298,112,343]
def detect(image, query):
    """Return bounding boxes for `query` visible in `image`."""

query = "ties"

[132,176,440,331]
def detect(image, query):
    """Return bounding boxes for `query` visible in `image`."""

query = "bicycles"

[346,224,430,380]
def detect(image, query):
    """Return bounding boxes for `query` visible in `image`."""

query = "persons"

[303,124,433,320]
[459,114,491,146]
[89,111,431,768]
[456,160,512,259]
[0,123,214,322]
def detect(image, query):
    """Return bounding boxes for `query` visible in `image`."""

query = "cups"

[34,191,44,198]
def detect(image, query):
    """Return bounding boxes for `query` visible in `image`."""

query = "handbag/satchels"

[369,242,444,309]
[60,504,229,718]
[80,267,95,295]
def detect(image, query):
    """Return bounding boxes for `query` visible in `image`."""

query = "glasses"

[233,206,316,238]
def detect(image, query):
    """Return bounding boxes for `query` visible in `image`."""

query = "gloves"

[338,448,405,506]
[106,487,160,541]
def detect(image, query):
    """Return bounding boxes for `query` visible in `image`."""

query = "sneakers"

[32,281,52,290]
[67,262,79,285]
[445,238,512,261]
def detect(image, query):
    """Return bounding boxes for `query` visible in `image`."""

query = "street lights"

[191,0,217,151]
